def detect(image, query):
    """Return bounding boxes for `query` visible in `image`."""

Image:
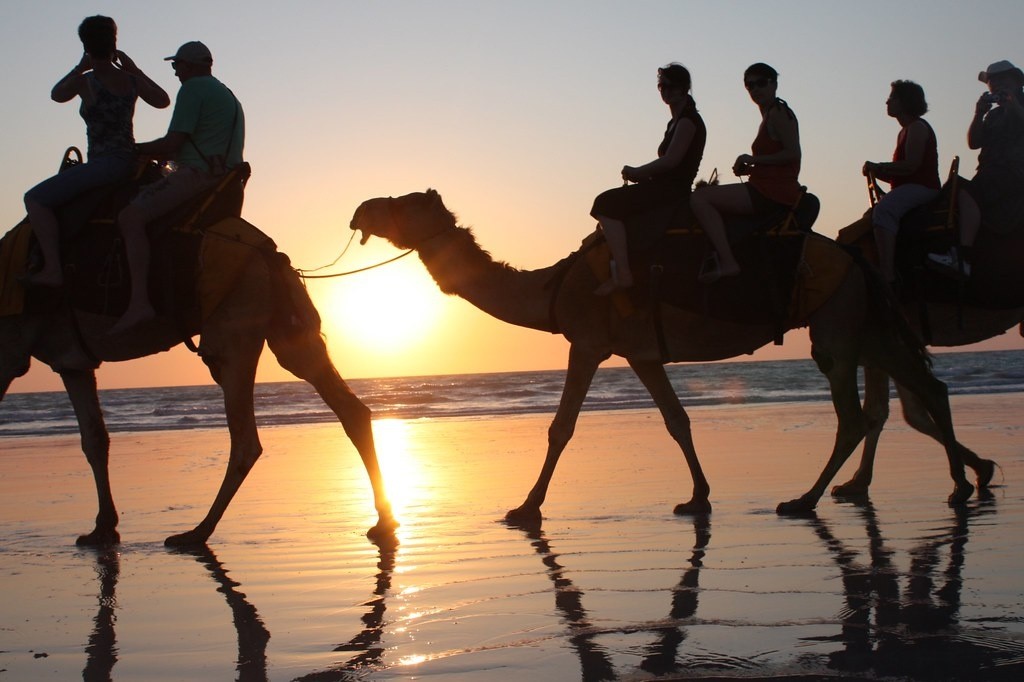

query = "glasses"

[658,81,683,92]
[171,61,181,69]
[745,78,772,90]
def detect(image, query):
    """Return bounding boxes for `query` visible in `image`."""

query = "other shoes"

[928,246,971,281]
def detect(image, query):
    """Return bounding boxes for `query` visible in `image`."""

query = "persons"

[689,62,801,276]
[589,61,706,298]
[23,14,171,287]
[861,80,943,292]
[106,41,252,338]
[953,59,1024,286]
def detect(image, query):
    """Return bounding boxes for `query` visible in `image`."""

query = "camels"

[349,170,1024,528]
[0,152,401,549]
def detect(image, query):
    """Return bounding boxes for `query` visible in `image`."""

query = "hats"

[977,60,1023,86]
[163,40,214,65]
